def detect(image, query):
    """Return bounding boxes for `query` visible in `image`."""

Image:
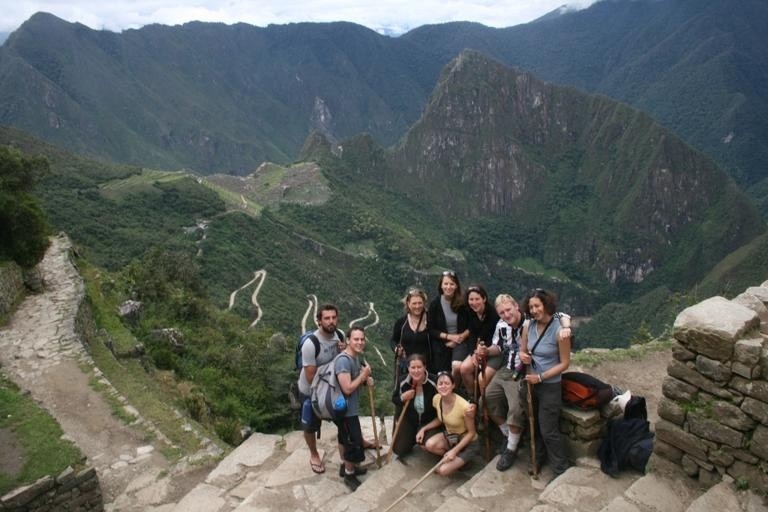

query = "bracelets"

[445,332,449,339]
[562,326,571,330]
[537,371,544,383]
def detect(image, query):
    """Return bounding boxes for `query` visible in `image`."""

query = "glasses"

[442,271,456,277]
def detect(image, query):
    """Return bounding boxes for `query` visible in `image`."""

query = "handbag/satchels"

[562,372,614,412]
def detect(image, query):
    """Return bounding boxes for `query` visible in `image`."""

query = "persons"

[519,288,572,486]
[296,303,383,473]
[391,288,432,376]
[331,327,374,491]
[476,292,574,471]
[426,269,471,369]
[460,285,502,427]
[413,369,479,476]
[392,353,478,459]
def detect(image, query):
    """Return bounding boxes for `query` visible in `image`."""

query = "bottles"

[301,397,312,426]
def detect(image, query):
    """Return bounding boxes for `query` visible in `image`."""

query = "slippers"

[364,443,383,450]
[309,458,325,474]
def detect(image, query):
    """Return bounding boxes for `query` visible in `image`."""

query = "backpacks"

[310,352,353,420]
[296,328,344,370]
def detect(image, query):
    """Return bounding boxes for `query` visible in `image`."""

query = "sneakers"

[529,463,541,476]
[459,461,472,472]
[340,464,367,492]
[496,436,518,471]
